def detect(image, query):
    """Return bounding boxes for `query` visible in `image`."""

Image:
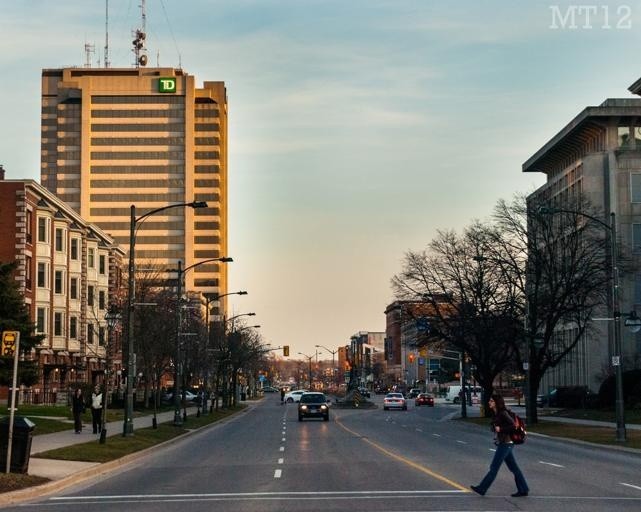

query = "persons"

[71,389,87,433]
[196,388,215,406]
[279,387,287,404]
[87,385,105,435]
[470,393,530,497]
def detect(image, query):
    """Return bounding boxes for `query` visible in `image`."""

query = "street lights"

[422,293,467,420]
[297,352,322,392]
[473,256,532,430]
[222,313,256,408]
[287,358,307,389]
[174,258,233,426]
[316,345,349,393]
[539,207,626,442]
[202,291,248,414]
[124,201,208,436]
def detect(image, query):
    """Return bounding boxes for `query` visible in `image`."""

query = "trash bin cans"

[7,385,20,411]
[0,417,36,474]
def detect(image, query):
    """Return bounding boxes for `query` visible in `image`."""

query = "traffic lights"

[409,353,414,363]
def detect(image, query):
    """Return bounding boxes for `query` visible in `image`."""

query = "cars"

[374,385,434,411]
[338,383,370,399]
[163,389,199,404]
[283,389,310,403]
[261,386,290,393]
[536,386,596,408]
[295,392,331,422]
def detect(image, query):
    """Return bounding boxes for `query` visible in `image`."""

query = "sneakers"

[471,485,484,495]
[510,492,528,498]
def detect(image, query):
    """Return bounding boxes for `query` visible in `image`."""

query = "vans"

[445,385,484,405]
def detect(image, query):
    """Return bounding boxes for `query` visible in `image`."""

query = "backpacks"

[501,408,527,444]
